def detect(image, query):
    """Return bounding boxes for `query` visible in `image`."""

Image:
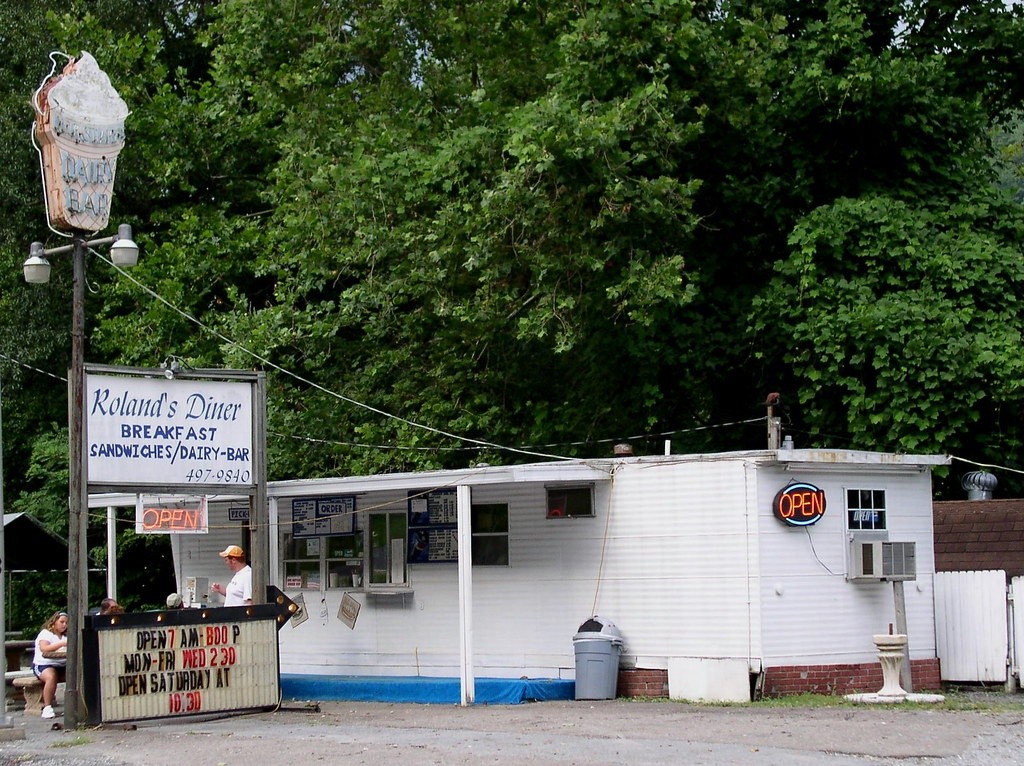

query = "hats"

[219,545,245,557]
[166,593,184,609]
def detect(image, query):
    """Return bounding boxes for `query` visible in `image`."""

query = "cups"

[352,572,360,587]
[330,573,338,587]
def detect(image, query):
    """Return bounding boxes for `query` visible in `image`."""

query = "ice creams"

[34,49,126,234]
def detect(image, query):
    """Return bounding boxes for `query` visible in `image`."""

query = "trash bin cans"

[572,616,624,700]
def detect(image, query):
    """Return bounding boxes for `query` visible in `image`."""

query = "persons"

[30,610,69,718]
[100,598,125,615]
[212,545,253,607]
[166,593,184,609]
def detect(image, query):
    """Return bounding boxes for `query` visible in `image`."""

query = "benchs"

[2,637,47,713]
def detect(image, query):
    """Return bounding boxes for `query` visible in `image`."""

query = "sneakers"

[41,705,55,718]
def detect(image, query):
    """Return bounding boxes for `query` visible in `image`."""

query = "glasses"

[110,606,118,609]
